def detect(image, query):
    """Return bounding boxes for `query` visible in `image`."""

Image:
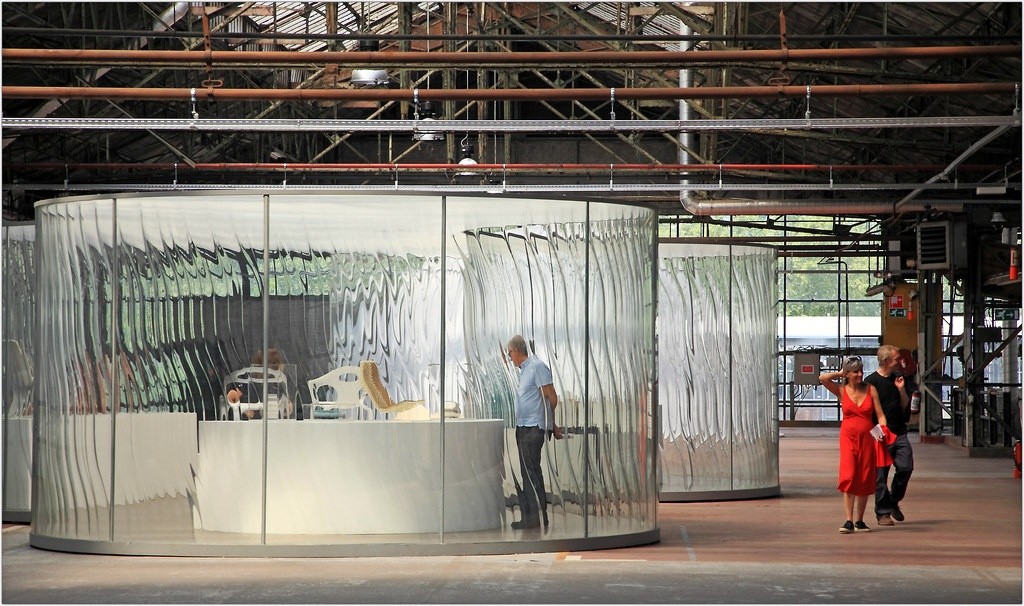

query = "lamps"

[413,2,445,141]
[350,2,390,85]
[453,8,486,177]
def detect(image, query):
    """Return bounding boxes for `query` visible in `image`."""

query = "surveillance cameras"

[991,212,1007,231]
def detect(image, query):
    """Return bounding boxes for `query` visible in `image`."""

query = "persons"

[865,345,915,526]
[509,335,566,529]
[818,355,888,532]
[228,349,302,421]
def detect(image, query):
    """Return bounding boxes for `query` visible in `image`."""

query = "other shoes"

[511,521,536,528]
[839,520,854,532]
[878,515,894,525]
[854,520,870,532]
[892,505,904,521]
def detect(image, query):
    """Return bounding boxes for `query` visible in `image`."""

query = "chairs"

[359,360,425,413]
[307,366,374,421]
[219,367,290,420]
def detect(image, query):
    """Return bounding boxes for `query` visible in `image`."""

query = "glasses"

[507,350,512,357]
[846,356,862,365]
